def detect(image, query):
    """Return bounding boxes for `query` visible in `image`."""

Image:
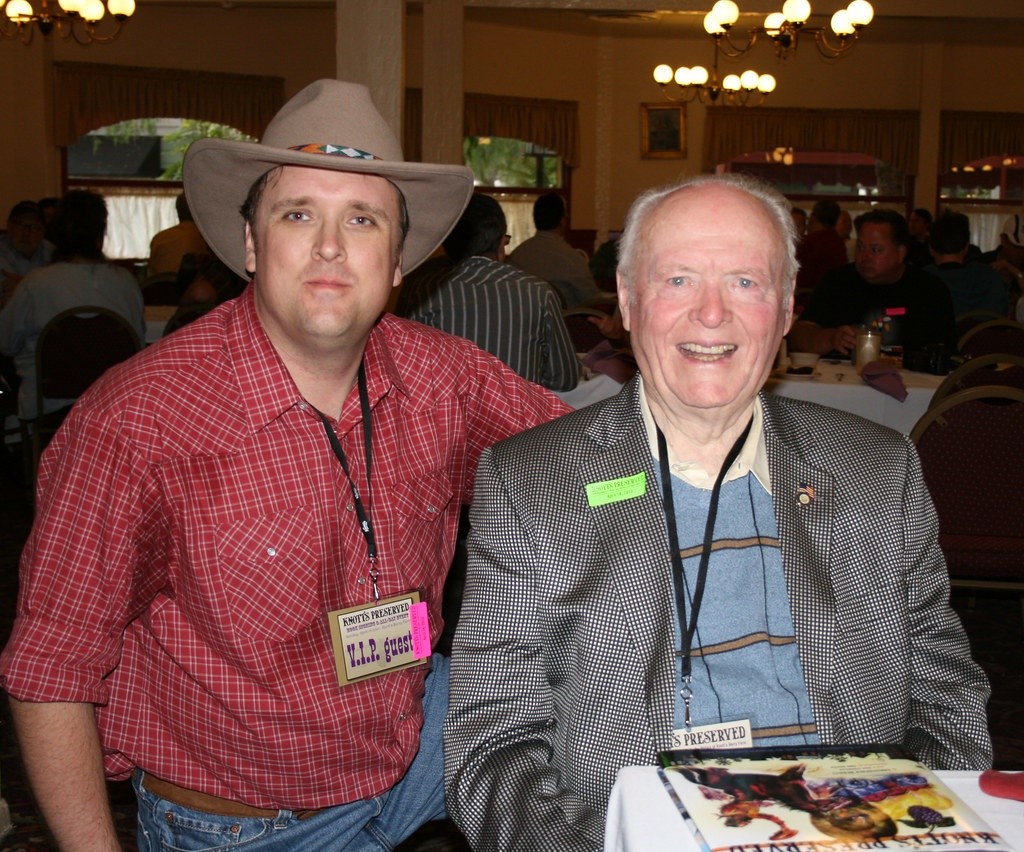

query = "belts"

[135,770,324,819]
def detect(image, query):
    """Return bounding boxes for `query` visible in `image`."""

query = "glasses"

[503,234,511,244]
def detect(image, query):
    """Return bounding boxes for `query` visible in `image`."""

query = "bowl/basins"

[790,352,819,367]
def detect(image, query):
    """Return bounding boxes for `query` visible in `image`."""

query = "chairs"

[0,257,249,520]
[544,249,1023,677]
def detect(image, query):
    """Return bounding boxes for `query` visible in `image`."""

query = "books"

[656,742,1016,852]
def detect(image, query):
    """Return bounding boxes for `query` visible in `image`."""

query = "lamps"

[703,1,874,66]
[653,37,776,115]
[0,0,135,45]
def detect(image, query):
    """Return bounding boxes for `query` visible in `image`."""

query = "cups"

[775,339,789,368]
[134,261,148,281]
[857,331,882,375]
[849,326,877,365]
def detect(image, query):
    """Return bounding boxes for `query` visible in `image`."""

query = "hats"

[181,80,476,281]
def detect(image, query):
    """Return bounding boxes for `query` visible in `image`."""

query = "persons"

[0,188,1024,443]
[445,170,997,852]
[0,79,579,852]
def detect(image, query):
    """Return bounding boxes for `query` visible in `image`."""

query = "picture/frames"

[636,101,686,162]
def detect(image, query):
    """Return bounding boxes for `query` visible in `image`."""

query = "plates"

[778,373,817,381]
